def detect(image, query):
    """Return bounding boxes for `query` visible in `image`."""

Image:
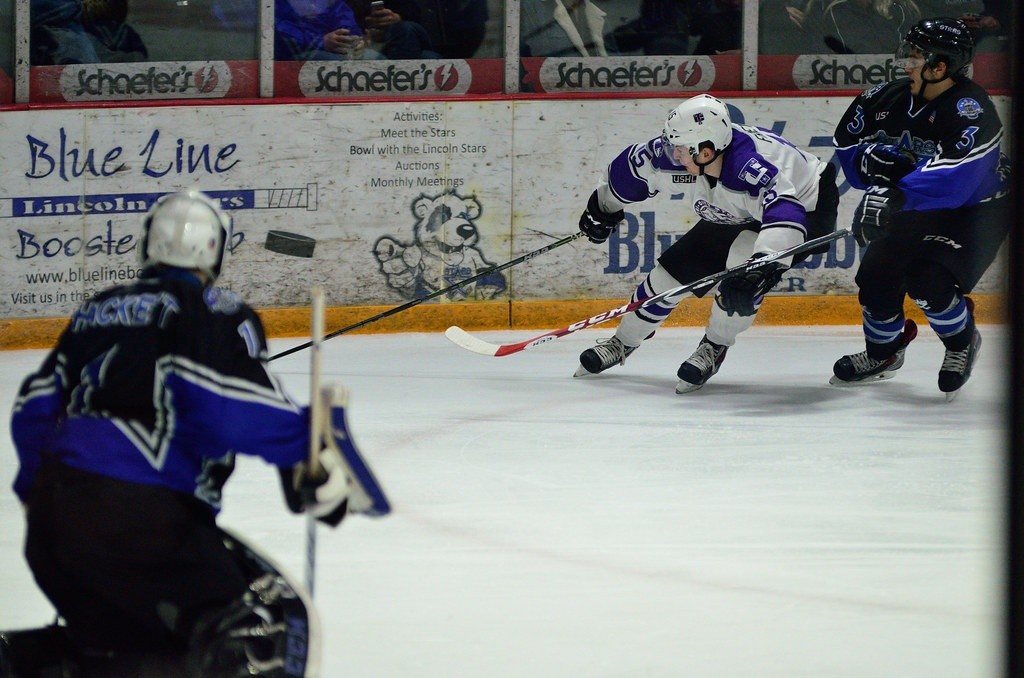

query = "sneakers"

[833,319,918,382]
[579,330,657,374]
[677,334,731,385]
[937,296,982,392]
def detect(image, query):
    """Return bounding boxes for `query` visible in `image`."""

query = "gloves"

[277,449,352,526]
[579,189,625,244]
[723,251,783,317]
[852,185,905,247]
[853,143,918,183]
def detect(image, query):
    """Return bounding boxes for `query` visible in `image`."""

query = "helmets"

[138,191,232,276]
[661,94,733,166]
[897,17,974,75]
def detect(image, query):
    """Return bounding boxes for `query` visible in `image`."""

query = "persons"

[0,190,392,678]
[0,0,147,67]
[573,94,840,394]
[520,0,1009,57]
[828,16,1012,403]
[211,0,488,60]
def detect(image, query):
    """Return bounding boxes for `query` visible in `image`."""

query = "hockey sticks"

[444,224,854,358]
[266,231,588,364]
[306,286,325,597]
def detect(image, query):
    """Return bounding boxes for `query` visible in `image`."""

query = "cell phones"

[371,1,384,12]
